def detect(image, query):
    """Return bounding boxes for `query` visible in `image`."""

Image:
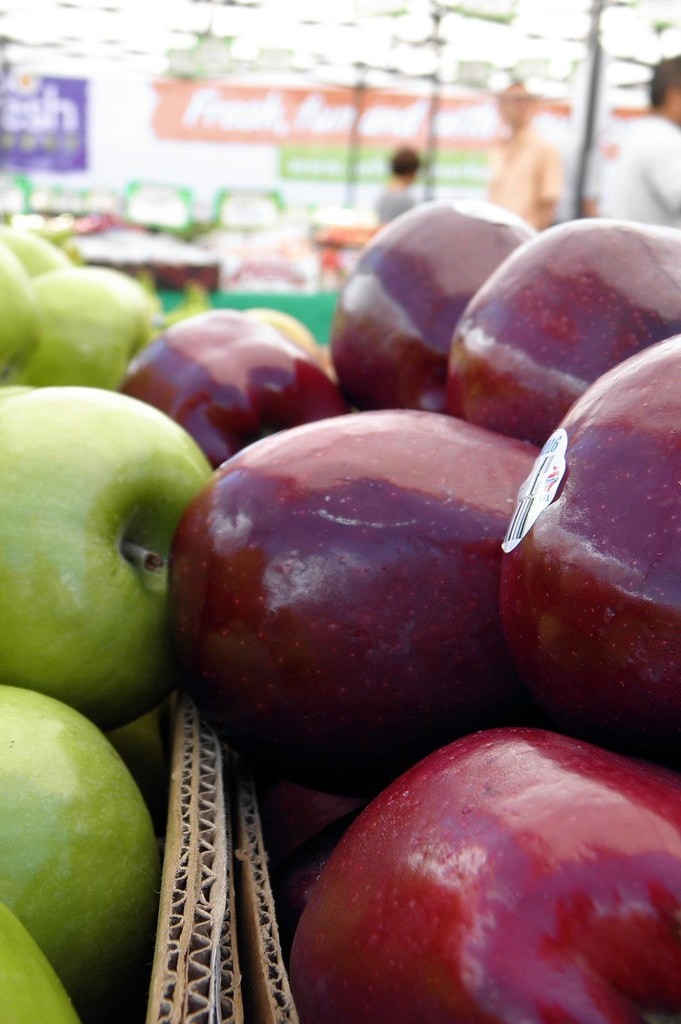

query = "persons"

[374,147,419,227]
[485,83,562,231]
[599,53,681,231]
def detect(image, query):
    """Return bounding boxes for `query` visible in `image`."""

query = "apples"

[0,194,681,1024]
